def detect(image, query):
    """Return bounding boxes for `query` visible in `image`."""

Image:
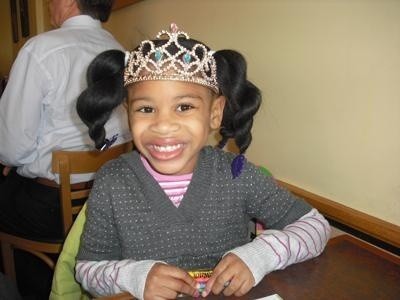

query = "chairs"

[0,140,136,297]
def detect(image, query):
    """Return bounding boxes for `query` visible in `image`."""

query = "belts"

[36,178,94,190]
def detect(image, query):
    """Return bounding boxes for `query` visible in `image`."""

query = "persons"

[1,1,131,300]
[74,26,332,300]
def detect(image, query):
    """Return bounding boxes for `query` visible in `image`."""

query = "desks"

[96,234,400,299]
